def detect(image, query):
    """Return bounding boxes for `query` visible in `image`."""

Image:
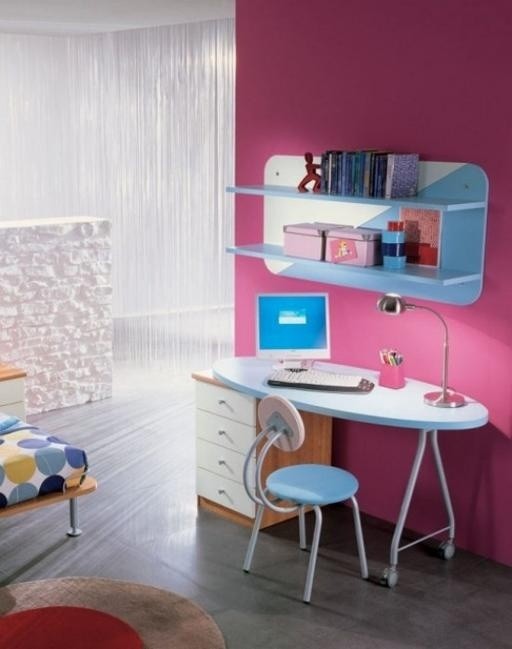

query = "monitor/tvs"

[255,292,331,370]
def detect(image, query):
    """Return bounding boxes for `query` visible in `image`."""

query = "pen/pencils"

[378,349,403,366]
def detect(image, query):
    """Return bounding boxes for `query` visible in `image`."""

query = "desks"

[212,356,490,589]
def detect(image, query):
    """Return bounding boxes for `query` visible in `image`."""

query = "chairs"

[241,394,370,604]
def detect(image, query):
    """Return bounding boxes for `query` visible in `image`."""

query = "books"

[317,148,420,199]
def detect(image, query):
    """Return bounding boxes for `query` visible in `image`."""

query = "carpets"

[0,575,226,649]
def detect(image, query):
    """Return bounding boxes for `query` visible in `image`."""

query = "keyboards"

[267,367,362,388]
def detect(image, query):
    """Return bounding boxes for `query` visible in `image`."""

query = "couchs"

[0,410,97,537]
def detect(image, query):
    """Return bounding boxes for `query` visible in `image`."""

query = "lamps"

[375,292,467,408]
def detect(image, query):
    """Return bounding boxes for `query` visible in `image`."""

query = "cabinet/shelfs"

[188,368,333,531]
[0,361,29,423]
[225,153,490,306]
